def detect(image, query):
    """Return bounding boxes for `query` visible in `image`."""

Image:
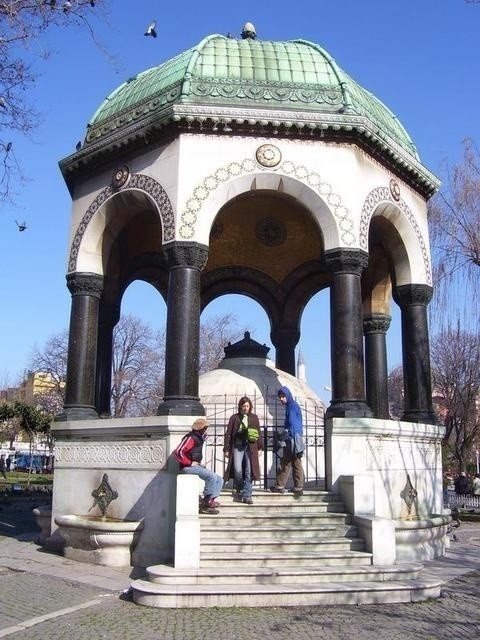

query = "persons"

[454,472,469,494]
[173,419,224,515]
[224,396,265,505]
[268,386,306,494]
[0,454,7,481]
[471,473,480,497]
[6,455,11,471]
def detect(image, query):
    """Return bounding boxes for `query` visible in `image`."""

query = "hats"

[192,418,210,431]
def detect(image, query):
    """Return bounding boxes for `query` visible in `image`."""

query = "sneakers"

[270,484,285,493]
[233,491,253,504]
[199,494,221,514]
[288,486,303,496]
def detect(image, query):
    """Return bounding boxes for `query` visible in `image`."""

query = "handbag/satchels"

[246,427,260,442]
